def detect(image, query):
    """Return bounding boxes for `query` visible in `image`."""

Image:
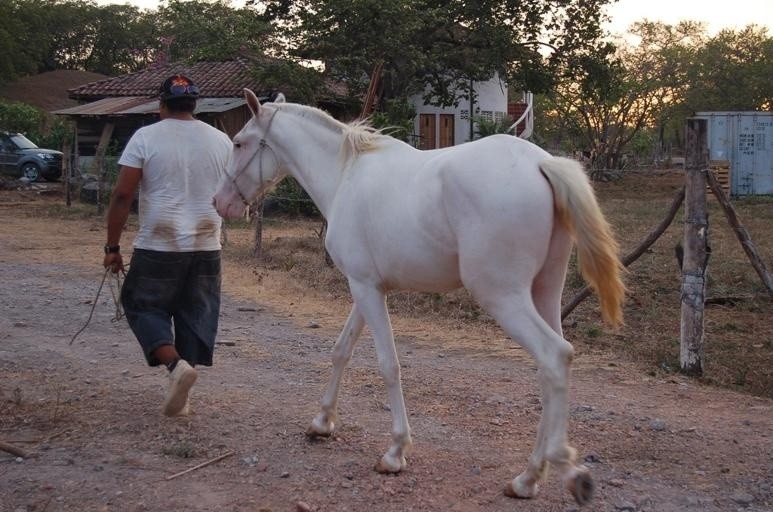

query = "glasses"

[160,84,200,97]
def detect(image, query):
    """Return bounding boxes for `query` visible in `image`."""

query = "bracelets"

[104,244,119,254]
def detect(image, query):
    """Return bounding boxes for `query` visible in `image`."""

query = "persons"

[101,73,234,420]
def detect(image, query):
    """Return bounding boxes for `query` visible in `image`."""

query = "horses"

[210,85,634,509]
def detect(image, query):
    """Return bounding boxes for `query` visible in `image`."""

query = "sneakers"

[164,357,198,418]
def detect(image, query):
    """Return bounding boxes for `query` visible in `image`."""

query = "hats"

[159,74,200,100]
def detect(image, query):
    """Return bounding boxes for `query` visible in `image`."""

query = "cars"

[0,130,64,182]
